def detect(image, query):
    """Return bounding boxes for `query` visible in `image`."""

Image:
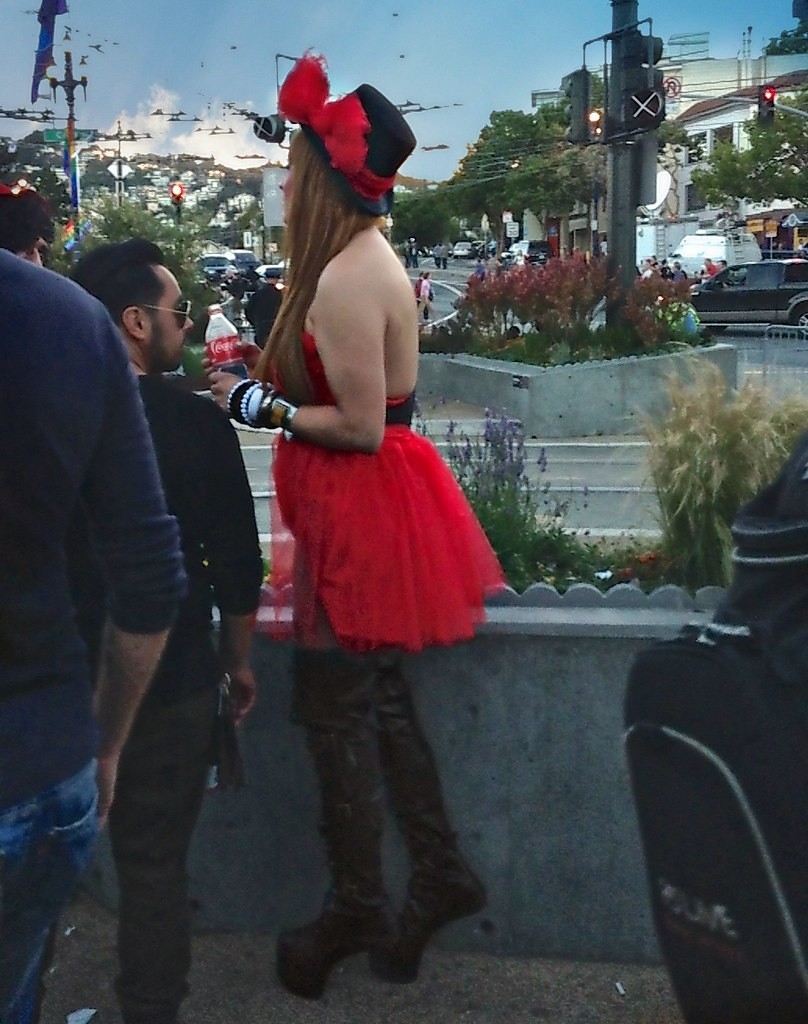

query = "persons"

[0,188,266,1024]
[574,246,584,263]
[210,45,506,999]
[414,272,434,322]
[797,239,804,250]
[601,235,607,254]
[503,255,513,270]
[495,258,508,276]
[245,269,284,346]
[204,265,224,286]
[474,259,485,280]
[242,265,262,283]
[403,238,419,268]
[641,255,688,280]
[512,250,527,266]
[227,272,248,301]
[694,258,722,284]
[433,243,448,270]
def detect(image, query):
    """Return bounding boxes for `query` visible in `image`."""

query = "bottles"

[206,304,251,382]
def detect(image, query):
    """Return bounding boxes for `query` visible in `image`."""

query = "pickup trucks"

[689,258,808,339]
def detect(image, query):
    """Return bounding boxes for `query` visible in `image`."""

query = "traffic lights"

[619,27,666,128]
[757,85,776,127]
[170,185,183,199]
[562,69,590,144]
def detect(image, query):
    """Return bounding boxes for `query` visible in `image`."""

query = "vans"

[665,228,764,282]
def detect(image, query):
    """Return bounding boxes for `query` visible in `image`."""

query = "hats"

[280,52,417,215]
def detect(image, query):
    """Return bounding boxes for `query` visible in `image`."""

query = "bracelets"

[227,379,303,431]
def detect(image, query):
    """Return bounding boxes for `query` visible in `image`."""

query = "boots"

[369,659,485,981]
[276,648,401,999]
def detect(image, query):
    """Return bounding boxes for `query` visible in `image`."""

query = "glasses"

[139,300,192,328]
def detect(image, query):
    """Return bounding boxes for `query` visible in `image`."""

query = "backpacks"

[623,425,808,1024]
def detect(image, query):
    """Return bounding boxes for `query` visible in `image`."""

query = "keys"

[216,674,231,718]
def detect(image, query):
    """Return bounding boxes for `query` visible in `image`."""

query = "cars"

[226,249,261,275]
[195,254,233,286]
[254,265,285,286]
[446,240,556,269]
